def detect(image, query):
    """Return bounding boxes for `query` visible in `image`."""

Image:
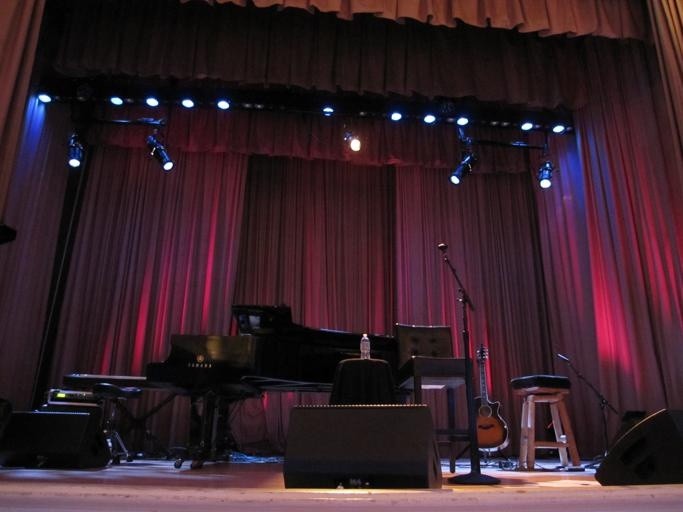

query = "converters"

[565,467,585,471]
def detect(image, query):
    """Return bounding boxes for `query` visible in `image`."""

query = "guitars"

[475,342,510,453]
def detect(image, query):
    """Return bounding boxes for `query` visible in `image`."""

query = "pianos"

[144,304,400,468]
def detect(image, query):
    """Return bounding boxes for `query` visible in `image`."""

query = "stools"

[512,386,580,470]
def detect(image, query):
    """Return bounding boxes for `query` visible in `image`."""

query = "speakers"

[47,404,104,467]
[594,408,683,486]
[0,411,94,469]
[283,404,442,489]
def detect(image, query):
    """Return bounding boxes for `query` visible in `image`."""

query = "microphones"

[557,354,568,360]
[438,244,448,262]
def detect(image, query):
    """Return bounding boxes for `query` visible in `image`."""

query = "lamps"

[344,132,360,151]
[144,135,174,172]
[66,135,83,168]
[448,150,480,185]
[535,159,554,190]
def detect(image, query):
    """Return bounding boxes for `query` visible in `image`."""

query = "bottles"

[359,333,371,360]
